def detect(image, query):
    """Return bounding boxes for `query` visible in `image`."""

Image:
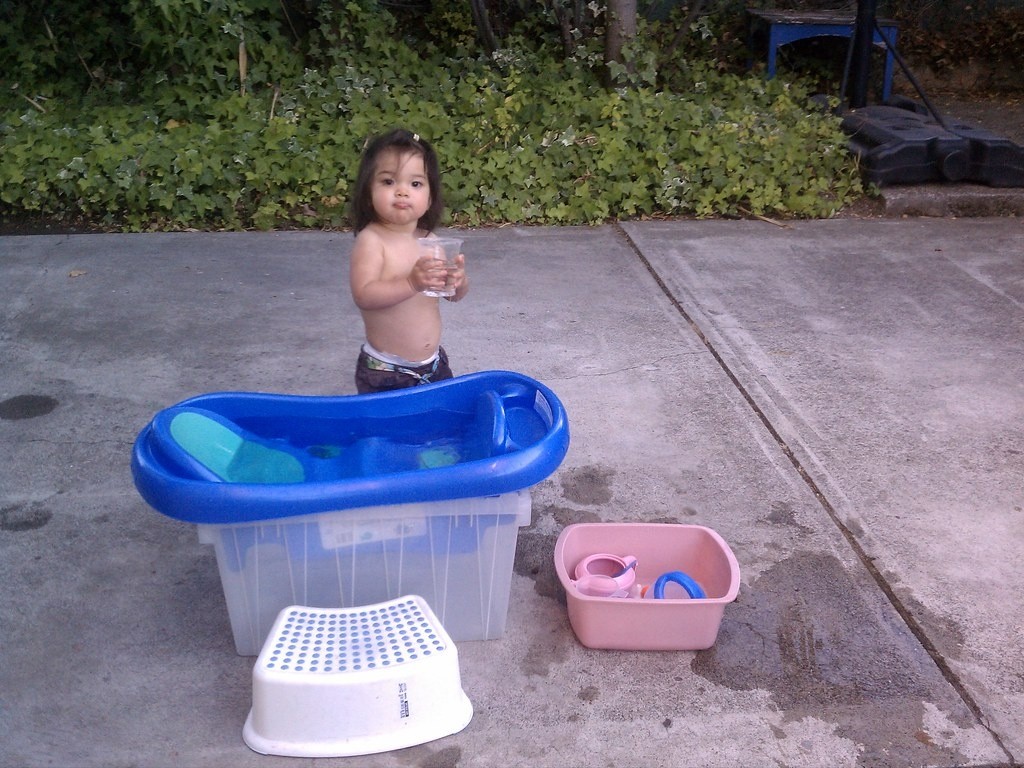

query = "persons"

[350,129,469,396]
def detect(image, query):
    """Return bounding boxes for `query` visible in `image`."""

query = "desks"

[745,7,901,106]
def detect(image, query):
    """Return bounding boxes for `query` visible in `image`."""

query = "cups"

[417,237,464,297]
[575,574,629,599]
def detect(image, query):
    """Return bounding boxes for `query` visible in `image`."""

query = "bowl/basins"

[642,571,706,599]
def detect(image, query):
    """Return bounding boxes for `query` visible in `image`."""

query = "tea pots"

[571,553,638,596]
